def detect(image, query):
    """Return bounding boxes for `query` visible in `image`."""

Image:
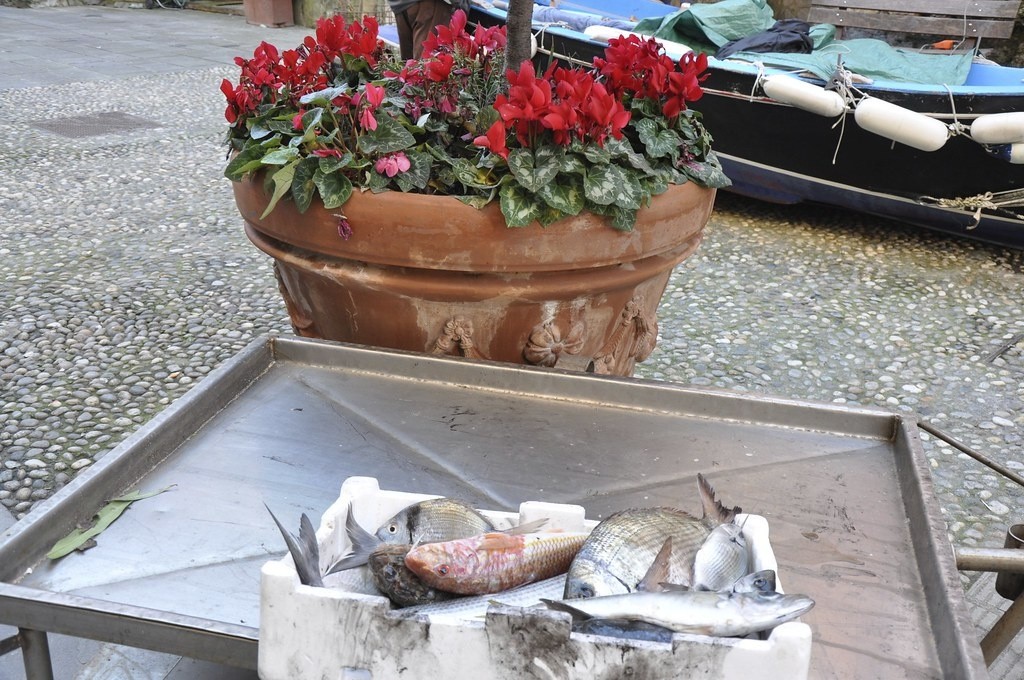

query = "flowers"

[212,7,735,235]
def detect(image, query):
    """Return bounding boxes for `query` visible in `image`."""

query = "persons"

[388,0,470,65]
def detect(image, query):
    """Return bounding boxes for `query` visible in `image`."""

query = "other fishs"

[265,474,815,642]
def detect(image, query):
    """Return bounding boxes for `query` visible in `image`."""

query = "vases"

[221,135,723,390]
